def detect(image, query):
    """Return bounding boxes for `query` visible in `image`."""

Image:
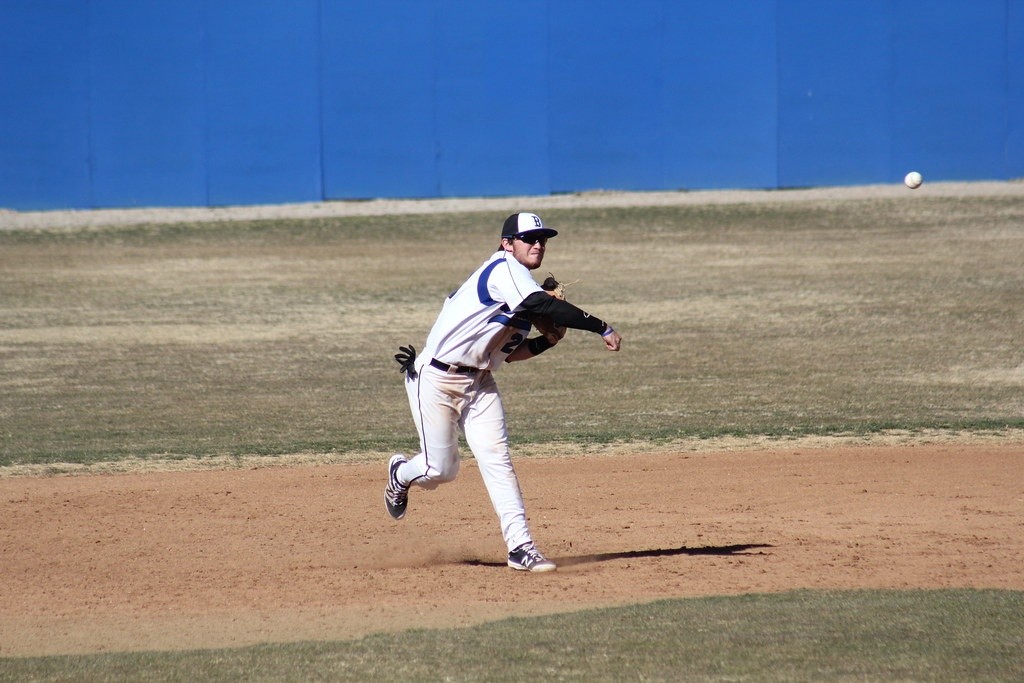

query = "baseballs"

[904,171,922,190]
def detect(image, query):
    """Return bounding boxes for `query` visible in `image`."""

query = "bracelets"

[601,325,614,337]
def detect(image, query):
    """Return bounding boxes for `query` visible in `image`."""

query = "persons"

[384,212,621,573]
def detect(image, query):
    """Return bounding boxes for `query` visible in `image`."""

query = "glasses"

[510,232,547,247]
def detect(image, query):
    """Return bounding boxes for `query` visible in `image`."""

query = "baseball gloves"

[533,277,566,335]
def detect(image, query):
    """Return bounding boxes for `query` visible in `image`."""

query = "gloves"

[394,344,416,380]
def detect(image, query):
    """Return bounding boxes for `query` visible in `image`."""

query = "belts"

[430,358,480,376]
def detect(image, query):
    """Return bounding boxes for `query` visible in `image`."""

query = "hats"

[501,213,558,238]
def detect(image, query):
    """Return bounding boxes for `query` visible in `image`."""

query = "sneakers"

[384,455,409,521]
[507,541,556,572]
